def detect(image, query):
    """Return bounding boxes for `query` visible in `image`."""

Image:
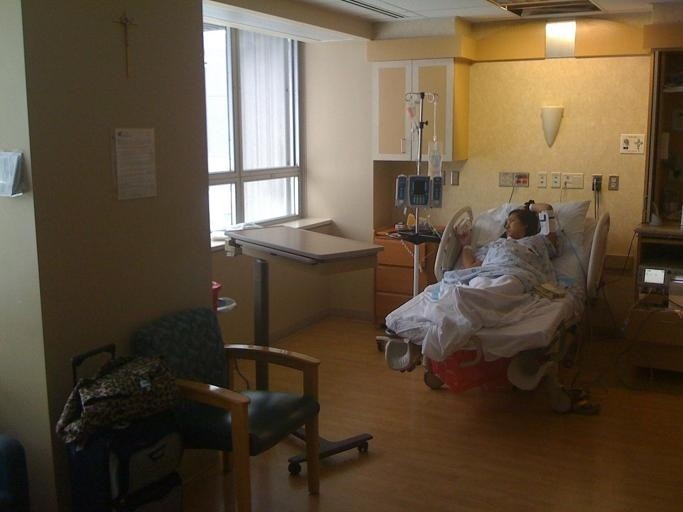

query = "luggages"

[65,341,185,512]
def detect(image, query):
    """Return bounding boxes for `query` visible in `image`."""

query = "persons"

[455,199,559,268]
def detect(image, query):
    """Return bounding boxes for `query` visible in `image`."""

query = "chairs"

[131,306,322,512]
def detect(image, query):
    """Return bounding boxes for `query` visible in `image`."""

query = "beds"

[384,198,610,413]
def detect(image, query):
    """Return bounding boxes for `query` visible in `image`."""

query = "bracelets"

[463,245,471,249]
[523,200,535,212]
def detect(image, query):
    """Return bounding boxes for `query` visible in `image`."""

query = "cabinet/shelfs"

[369,59,470,163]
[628,23,683,380]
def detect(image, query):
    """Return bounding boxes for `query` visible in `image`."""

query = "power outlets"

[608,176,619,190]
[513,172,529,187]
[592,174,602,192]
[537,172,547,188]
[552,172,561,188]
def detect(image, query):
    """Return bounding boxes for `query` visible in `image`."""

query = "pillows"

[500,199,591,252]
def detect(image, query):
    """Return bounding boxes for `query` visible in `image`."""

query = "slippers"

[561,384,604,417]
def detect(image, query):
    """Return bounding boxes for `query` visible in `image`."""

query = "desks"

[222,224,384,475]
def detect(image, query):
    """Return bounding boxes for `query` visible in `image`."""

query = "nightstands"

[373,226,444,322]
